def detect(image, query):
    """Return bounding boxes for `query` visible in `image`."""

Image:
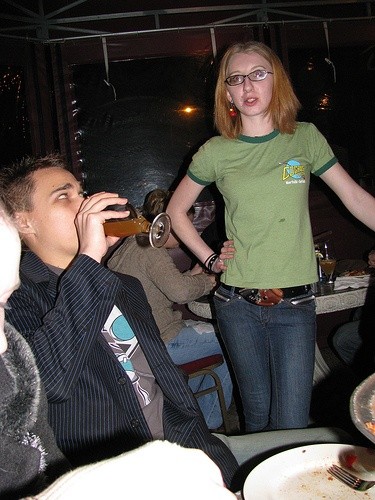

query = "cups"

[318,242,336,284]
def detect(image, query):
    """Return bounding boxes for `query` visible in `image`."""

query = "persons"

[190,222,332,389]
[333,307,375,366]
[166,42,375,434]
[108,188,233,434]
[0,195,71,500]
[0,150,245,500]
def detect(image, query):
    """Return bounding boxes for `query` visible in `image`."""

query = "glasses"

[225,69,273,86]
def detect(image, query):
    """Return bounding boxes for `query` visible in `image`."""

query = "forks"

[327,465,375,491]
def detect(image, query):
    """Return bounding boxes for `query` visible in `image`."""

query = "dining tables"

[310,285,369,390]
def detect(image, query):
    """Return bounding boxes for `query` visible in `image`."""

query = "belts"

[222,283,316,306]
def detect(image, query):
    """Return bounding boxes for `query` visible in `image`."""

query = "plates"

[243,443,375,500]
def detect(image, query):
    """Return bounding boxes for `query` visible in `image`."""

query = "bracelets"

[204,252,219,272]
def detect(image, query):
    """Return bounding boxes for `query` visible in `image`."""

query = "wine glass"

[102,201,171,249]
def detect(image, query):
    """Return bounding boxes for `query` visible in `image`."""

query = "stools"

[178,354,232,436]
